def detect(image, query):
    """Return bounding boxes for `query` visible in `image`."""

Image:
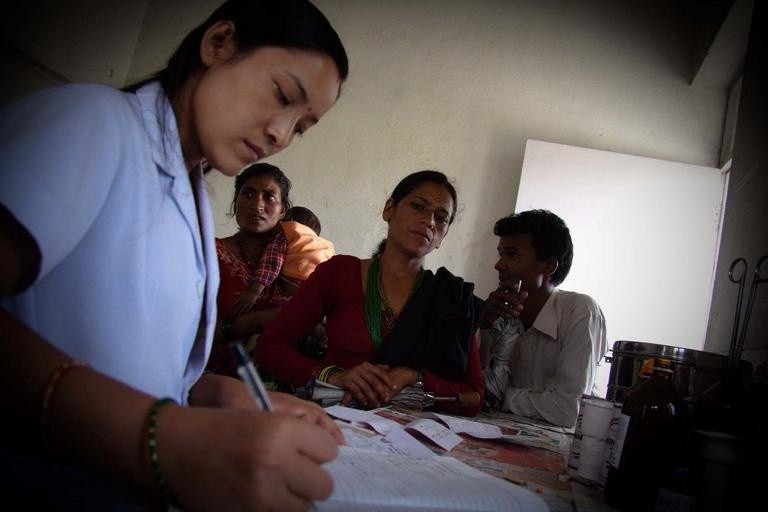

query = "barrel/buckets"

[608,340,752,434]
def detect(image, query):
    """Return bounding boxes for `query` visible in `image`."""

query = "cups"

[576,394,614,483]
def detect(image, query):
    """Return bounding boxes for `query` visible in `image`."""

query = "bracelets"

[318,365,344,383]
[37,360,90,428]
[417,373,424,387]
[148,397,179,501]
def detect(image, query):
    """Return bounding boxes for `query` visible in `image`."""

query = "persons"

[0,1,346,511]
[204,163,327,393]
[480,210,606,428]
[229,207,329,316]
[256,170,486,417]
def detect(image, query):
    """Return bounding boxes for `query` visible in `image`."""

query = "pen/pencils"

[229,339,271,413]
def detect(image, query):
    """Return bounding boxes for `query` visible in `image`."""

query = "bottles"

[601,358,688,512]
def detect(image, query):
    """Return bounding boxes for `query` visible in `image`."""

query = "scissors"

[726,255,767,357]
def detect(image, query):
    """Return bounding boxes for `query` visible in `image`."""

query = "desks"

[296,402,618,511]
